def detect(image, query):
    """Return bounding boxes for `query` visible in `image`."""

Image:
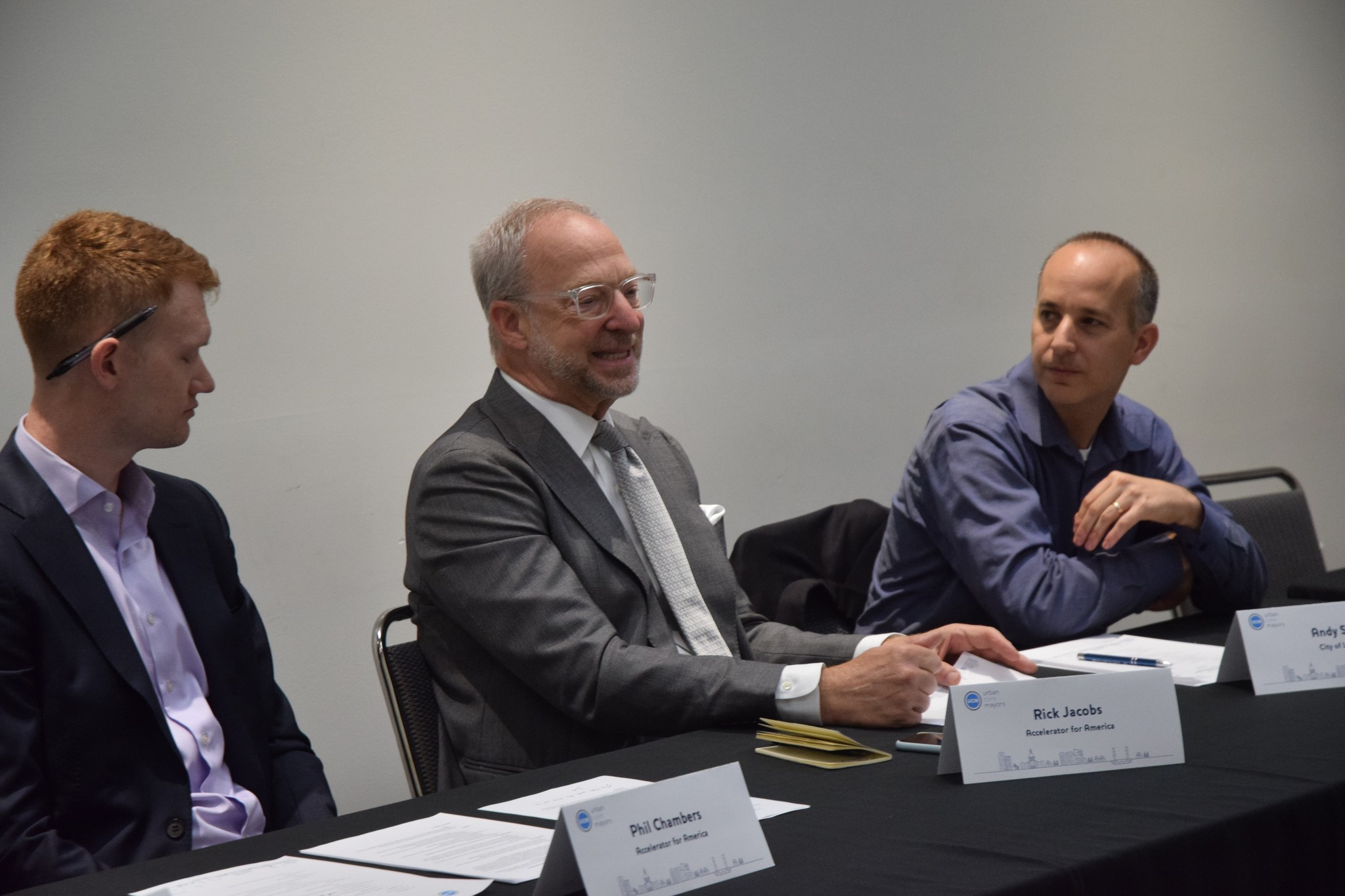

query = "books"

[751,717,892,770]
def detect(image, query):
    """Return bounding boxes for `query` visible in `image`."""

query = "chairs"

[373,606,439,797]
[1172,465,1324,617]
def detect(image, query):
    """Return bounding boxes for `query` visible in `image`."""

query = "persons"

[401,196,1037,796]
[0,212,337,885]
[846,229,1266,658]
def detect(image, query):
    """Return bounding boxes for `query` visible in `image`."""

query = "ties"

[590,419,733,656]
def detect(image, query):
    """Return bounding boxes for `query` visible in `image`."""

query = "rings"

[1112,502,1123,515]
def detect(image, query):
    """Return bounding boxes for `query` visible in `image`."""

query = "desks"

[26,568,1345,896]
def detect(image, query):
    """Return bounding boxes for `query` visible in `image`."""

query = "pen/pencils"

[1078,653,1172,668]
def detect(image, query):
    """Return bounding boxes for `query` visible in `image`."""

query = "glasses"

[503,272,656,321]
